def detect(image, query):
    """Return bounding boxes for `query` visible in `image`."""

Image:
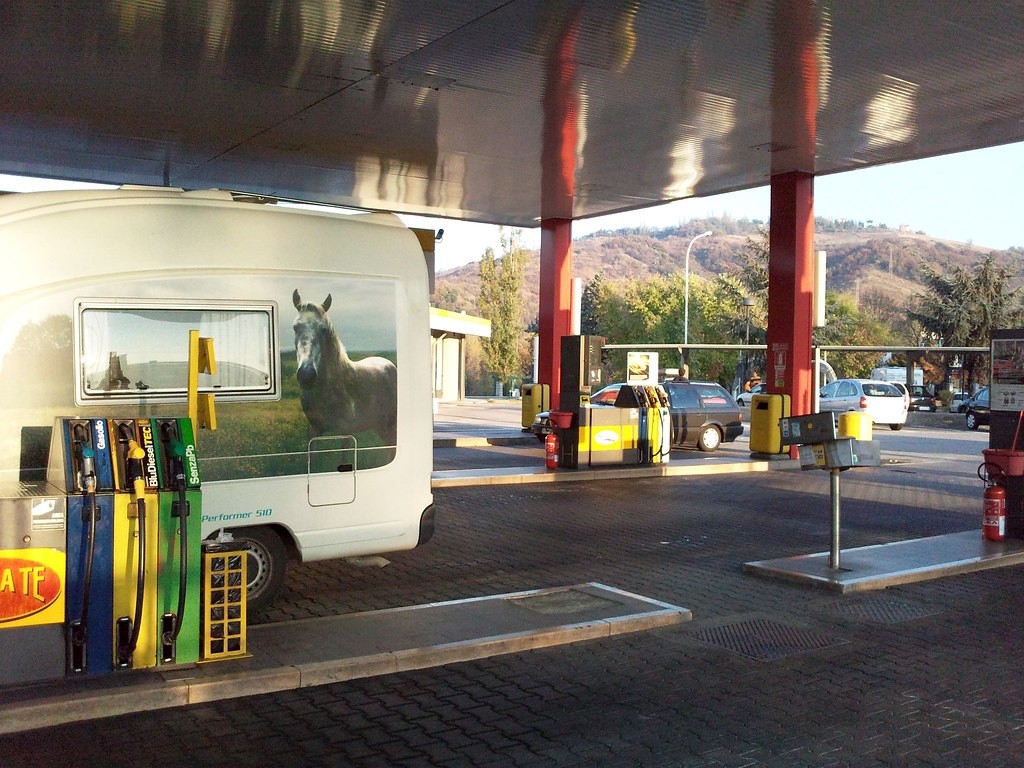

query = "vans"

[0,184,435,616]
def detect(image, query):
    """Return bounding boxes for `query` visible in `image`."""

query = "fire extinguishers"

[542,420,562,470]
[976,461,1011,542]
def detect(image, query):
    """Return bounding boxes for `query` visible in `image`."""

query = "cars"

[736,383,766,406]
[950,393,969,413]
[966,387,990,430]
[903,384,936,412]
[531,383,744,452]
[820,379,910,430]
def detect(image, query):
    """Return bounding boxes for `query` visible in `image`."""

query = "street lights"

[743,296,754,379]
[685,231,713,344]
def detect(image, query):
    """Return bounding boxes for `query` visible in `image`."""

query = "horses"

[291,288,398,465]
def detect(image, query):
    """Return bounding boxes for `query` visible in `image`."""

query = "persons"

[673,367,688,382]
[744,370,762,391]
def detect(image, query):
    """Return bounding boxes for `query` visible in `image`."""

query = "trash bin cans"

[749,394,791,454]
[520,383,551,432]
